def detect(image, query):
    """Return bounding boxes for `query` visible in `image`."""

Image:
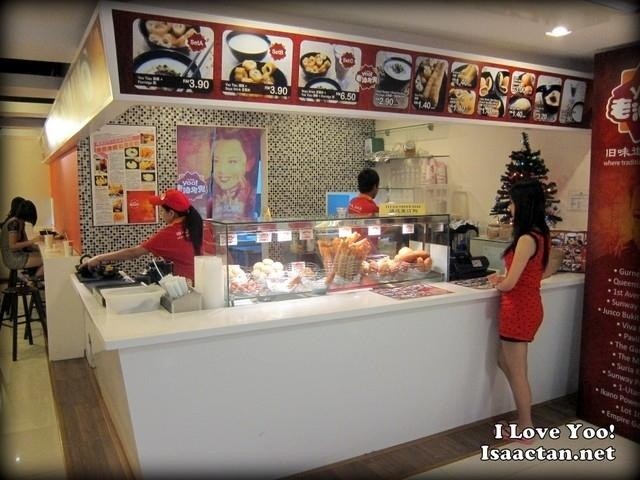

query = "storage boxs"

[100,284,165,314]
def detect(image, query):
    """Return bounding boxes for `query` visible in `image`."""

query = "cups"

[44,235,53,253]
[64,241,73,256]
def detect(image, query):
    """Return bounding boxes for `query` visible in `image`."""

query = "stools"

[0,267,48,361]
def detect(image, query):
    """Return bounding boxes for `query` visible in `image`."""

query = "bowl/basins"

[130,15,584,123]
[39,230,58,236]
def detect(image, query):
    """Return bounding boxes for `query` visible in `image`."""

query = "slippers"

[493,427,536,443]
[497,418,520,427]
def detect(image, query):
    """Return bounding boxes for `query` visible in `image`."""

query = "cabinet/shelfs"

[360,155,449,216]
[203,213,452,308]
[469,234,512,275]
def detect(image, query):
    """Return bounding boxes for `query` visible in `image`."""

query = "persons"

[192,128,256,219]
[489,178,551,444]
[2,200,45,288]
[77,189,215,286]
[346,168,390,256]
[0,197,29,243]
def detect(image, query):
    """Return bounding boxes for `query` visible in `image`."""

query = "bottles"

[403,140,416,157]
[487,221,513,243]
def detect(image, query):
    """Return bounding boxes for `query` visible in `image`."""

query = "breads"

[317,232,372,285]
[394,247,432,271]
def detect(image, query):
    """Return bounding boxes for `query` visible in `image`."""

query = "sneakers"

[27,276,44,289]
[17,271,28,282]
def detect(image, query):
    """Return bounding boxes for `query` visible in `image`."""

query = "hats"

[147,189,191,213]
